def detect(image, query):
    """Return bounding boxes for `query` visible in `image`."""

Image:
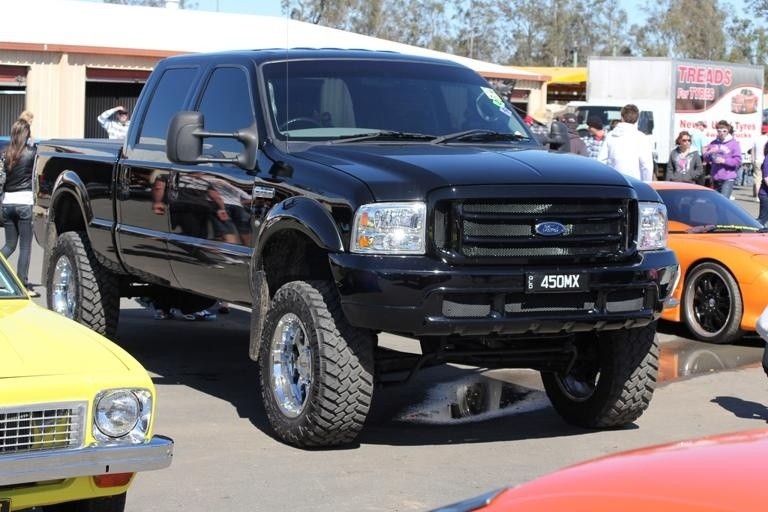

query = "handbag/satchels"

[0,153,7,195]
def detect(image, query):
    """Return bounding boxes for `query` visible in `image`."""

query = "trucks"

[566,54,765,195]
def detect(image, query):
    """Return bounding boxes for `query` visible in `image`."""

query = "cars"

[421,428,768,512]
[0,137,47,228]
[730,89,759,113]
[654,335,757,381]
[0,250,175,512]
[639,180,768,345]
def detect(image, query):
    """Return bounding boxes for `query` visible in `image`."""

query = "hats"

[562,112,577,129]
[587,116,603,129]
[529,109,548,125]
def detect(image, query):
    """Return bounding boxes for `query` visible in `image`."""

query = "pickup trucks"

[31,46,680,451]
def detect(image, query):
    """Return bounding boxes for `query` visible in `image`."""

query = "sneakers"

[28,288,39,297]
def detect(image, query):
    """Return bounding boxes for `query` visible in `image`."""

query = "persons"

[152,169,254,269]
[528,103,768,226]
[0,109,42,298]
[97,105,132,141]
